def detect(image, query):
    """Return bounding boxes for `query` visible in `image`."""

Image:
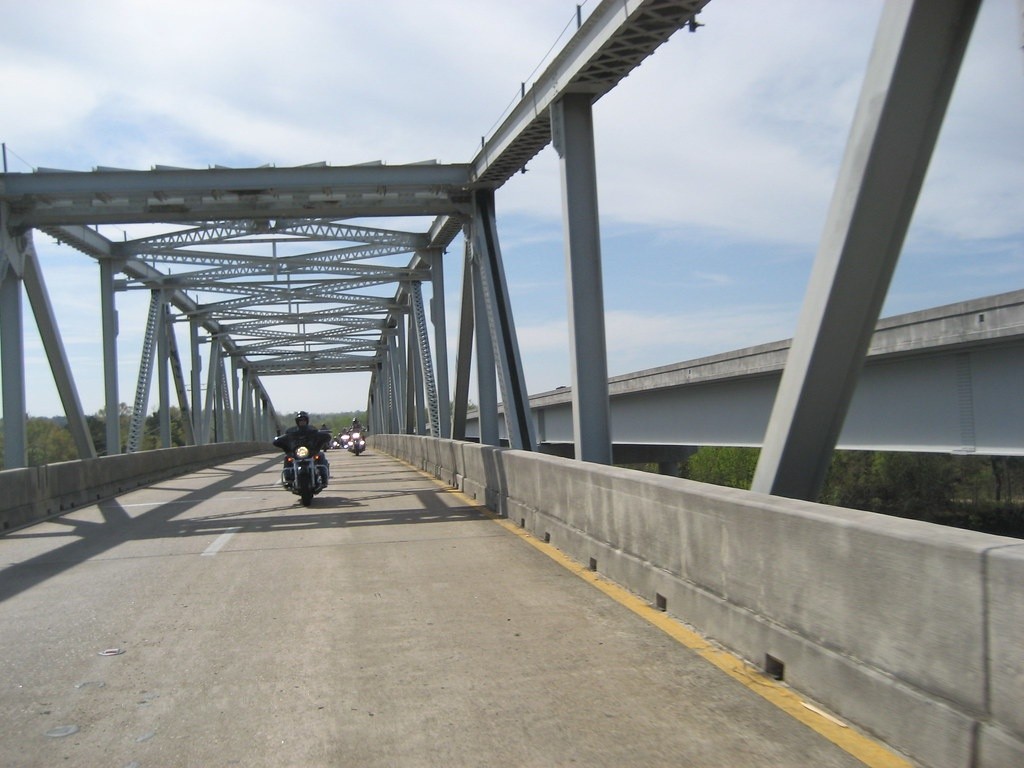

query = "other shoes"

[284,483,291,487]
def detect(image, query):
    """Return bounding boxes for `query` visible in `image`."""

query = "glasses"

[299,419,306,421]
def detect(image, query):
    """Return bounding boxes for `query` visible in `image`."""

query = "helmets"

[295,411,308,425]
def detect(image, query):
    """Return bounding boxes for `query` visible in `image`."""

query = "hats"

[352,417,358,421]
[321,423,325,426]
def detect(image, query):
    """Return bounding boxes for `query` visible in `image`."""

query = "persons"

[319,416,367,452]
[282,410,330,487]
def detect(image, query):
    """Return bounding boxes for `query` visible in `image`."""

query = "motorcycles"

[333,428,367,456]
[279,438,330,507]
